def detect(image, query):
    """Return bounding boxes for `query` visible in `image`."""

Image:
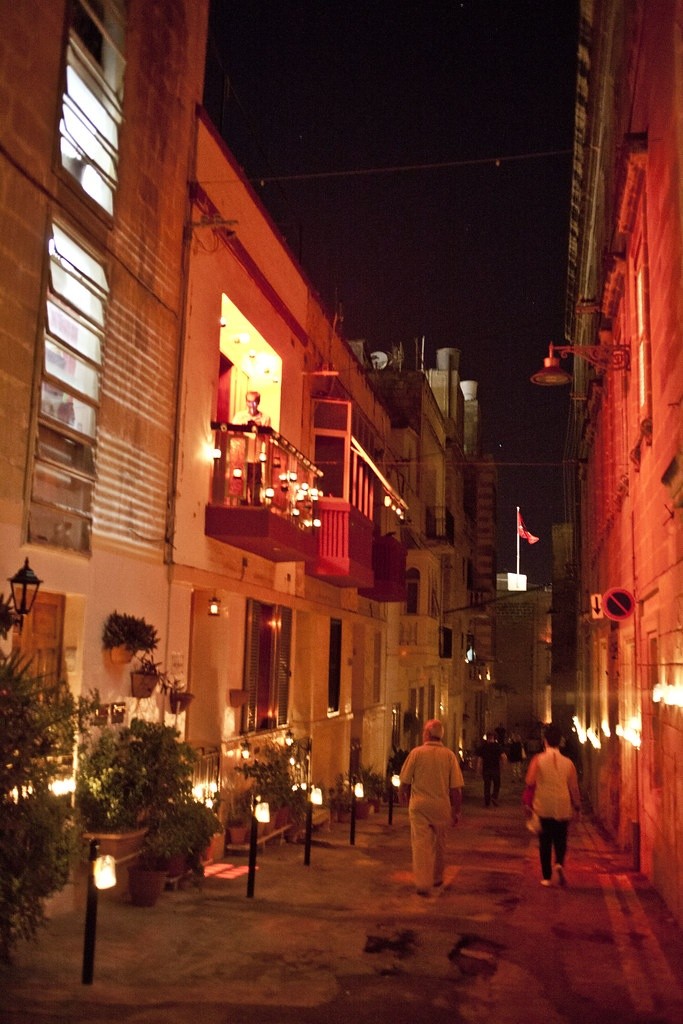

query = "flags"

[516,510,540,545]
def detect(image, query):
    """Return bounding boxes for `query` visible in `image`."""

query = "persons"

[397,718,466,897]
[227,391,272,507]
[473,707,582,809]
[521,723,583,887]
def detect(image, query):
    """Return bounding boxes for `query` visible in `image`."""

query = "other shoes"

[555,864,567,886]
[416,886,429,896]
[541,879,552,886]
[433,880,444,887]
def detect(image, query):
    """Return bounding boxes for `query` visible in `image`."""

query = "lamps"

[210,438,323,532]
